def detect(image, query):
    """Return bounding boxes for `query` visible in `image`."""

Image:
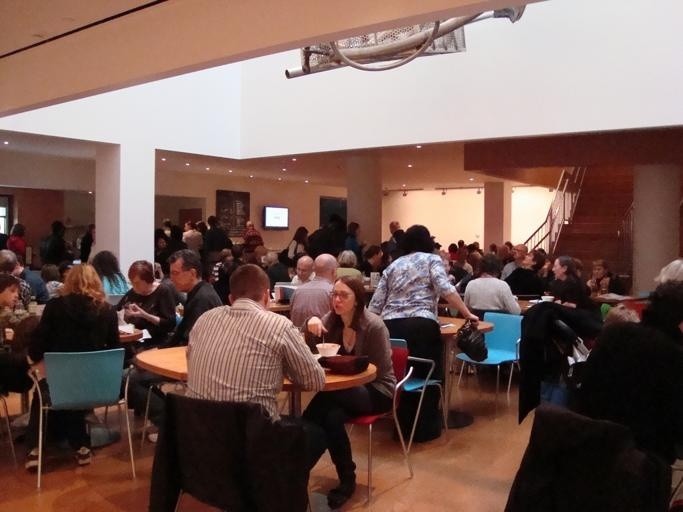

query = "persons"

[0,214,683,444]
[184,262,329,475]
[299,277,397,508]
[25,261,122,473]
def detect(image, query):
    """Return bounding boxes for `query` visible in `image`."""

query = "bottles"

[27,295,38,316]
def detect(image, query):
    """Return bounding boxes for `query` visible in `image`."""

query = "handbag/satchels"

[457,319,488,362]
[279,248,305,266]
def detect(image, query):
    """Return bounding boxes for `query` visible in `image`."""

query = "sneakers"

[26,447,40,469]
[74,447,91,465]
[148,432,158,443]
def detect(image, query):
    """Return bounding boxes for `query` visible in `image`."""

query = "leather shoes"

[328,483,355,509]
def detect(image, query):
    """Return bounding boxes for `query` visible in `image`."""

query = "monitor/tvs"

[264,206,290,231]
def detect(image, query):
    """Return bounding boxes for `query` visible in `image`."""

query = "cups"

[370,271,380,288]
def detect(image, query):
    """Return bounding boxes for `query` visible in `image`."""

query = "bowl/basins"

[541,295,555,302]
[316,343,341,358]
